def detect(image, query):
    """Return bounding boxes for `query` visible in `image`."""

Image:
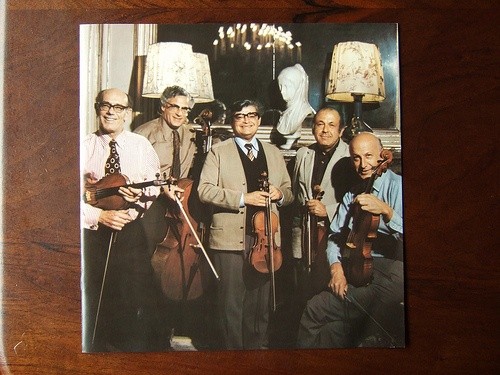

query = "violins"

[341,150,393,287]
[248,172,284,274]
[83,174,177,211]
[302,185,329,274]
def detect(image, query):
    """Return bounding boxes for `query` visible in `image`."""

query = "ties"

[171,131,181,179]
[244,144,255,161]
[104,140,121,177]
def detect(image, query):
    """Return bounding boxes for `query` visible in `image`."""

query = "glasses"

[234,112,259,120]
[97,102,129,113]
[166,101,191,114]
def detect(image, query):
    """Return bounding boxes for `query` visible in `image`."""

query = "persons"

[281,107,364,348]
[80,88,163,351]
[132,85,199,261]
[275,62,315,136]
[293,130,405,348]
[195,97,296,348]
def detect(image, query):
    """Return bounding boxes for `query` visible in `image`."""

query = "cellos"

[151,108,212,302]
